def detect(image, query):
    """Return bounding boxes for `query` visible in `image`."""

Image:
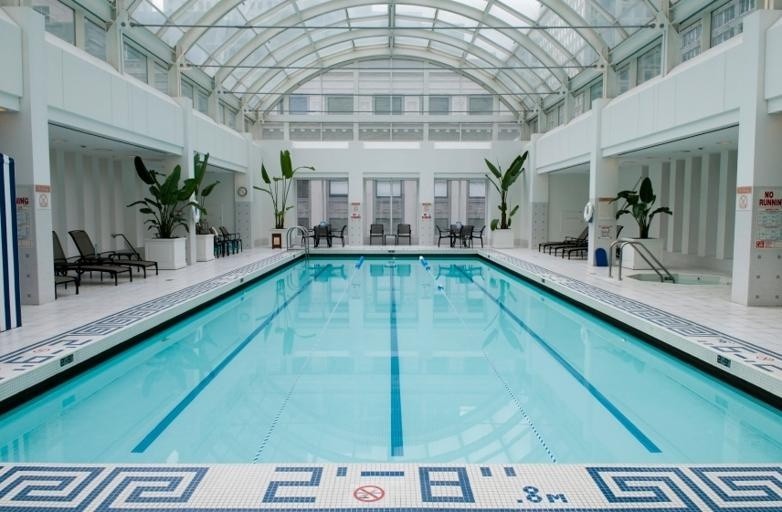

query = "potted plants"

[253,148,315,250]
[608,176,673,270]
[484,148,528,248]
[126,150,220,270]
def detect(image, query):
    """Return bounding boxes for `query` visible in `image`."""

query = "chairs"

[538,225,624,260]
[52,230,158,300]
[370,224,386,245]
[209,226,242,259]
[395,224,411,244]
[435,225,486,248]
[300,223,347,248]
